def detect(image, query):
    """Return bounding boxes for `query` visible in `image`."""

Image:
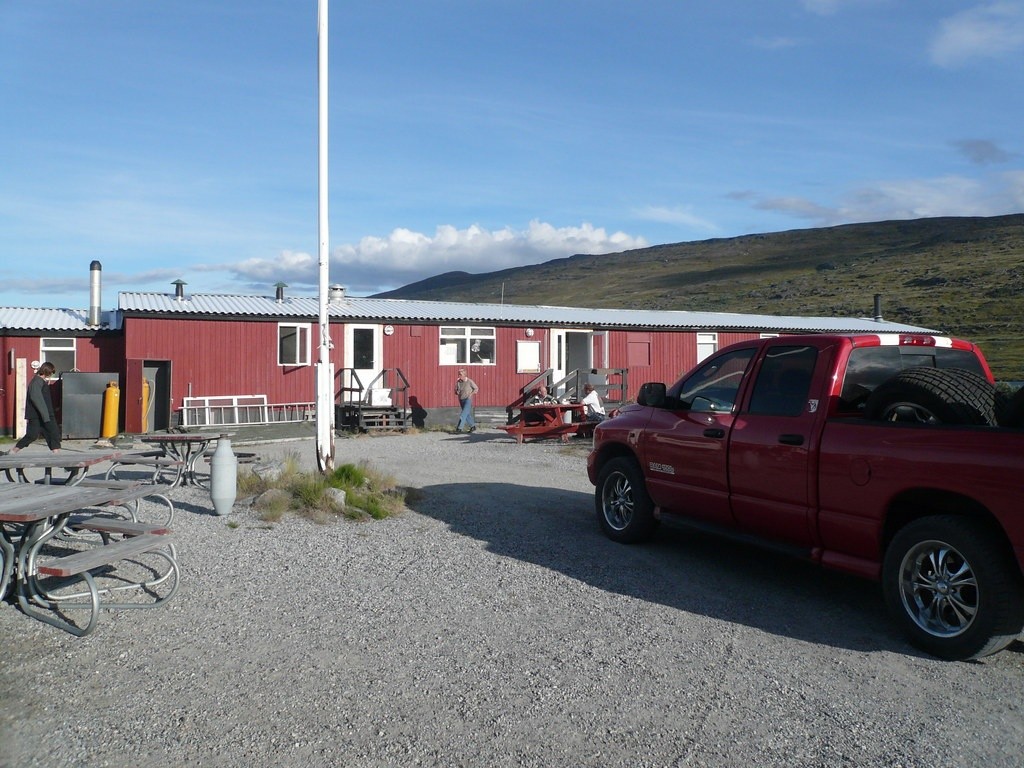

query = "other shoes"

[456,428,463,432]
[572,434,584,438]
[469,426,476,433]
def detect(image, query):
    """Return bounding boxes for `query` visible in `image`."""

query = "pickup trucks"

[587,334,1023,661]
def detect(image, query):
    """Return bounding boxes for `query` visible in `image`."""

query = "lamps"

[527,328,534,337]
[385,325,394,336]
[30,360,40,373]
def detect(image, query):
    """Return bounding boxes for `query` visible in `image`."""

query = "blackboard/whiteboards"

[517,340,541,373]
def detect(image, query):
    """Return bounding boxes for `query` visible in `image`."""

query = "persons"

[454,368,478,433]
[573,383,606,438]
[8,362,61,454]
[527,386,557,406]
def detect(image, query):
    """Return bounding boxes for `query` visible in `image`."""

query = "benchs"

[496,421,603,446]
[0,447,261,637]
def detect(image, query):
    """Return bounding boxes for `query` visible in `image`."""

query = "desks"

[140,432,236,491]
[0,452,124,538]
[0,483,123,637]
[515,404,584,445]
